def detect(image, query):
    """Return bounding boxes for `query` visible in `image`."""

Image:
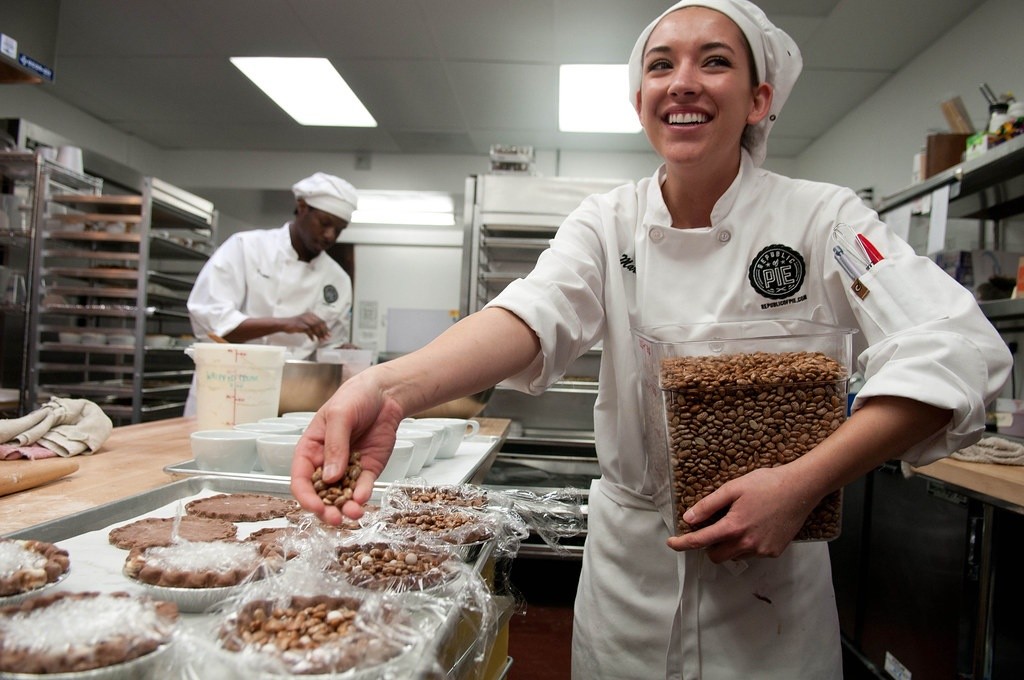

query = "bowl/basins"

[278,360,344,417]
[189,411,480,482]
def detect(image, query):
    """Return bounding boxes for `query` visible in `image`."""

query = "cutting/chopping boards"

[0,416,194,536]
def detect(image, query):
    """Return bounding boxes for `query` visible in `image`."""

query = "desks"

[0,417,512,536]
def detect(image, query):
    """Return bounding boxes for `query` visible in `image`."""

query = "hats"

[291,172,357,222]
[628,0,803,168]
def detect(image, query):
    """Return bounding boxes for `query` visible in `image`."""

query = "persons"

[291,1,1013,679]
[184,171,359,417]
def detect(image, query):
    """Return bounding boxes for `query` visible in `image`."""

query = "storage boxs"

[632,318,860,552]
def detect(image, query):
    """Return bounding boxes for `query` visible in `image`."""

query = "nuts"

[311,451,362,505]
[659,354,847,541]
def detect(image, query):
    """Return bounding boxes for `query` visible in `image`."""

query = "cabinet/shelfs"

[459,173,624,557]
[1,152,218,423]
[829,134,1023,679]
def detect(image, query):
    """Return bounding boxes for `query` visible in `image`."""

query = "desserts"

[0,490,500,673]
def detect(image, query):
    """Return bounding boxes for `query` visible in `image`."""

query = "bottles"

[911,144,927,184]
